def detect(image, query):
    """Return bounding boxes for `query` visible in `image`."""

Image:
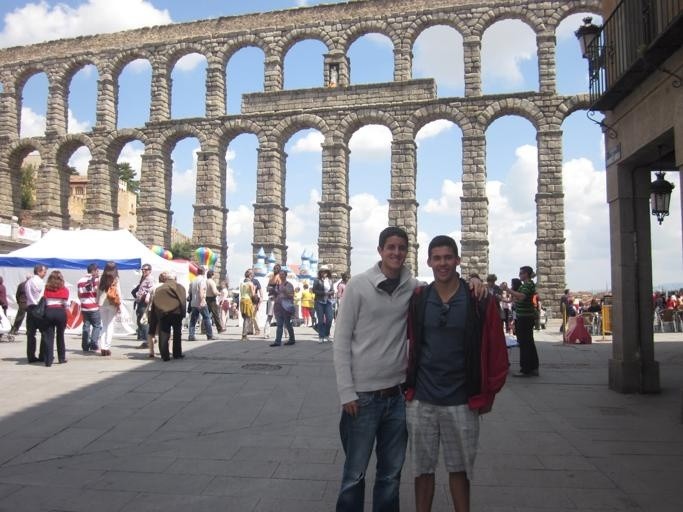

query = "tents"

[0,227,191,335]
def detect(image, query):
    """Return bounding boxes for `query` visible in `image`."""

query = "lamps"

[574,16,606,72]
[649,145,674,225]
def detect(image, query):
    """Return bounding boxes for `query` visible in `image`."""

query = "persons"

[403,235,511,512]
[1,278,7,313]
[7,275,32,342]
[187,264,349,347]
[486,266,683,377]
[78,261,122,356]
[334,228,489,511]
[135,264,187,362]
[24,264,54,363]
[42,271,69,367]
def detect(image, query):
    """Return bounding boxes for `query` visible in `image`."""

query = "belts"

[372,384,406,399]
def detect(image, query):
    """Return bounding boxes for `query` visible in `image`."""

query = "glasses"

[437,303,450,330]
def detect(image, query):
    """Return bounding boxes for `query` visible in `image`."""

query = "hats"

[319,264,330,271]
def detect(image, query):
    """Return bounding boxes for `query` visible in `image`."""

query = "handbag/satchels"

[281,298,294,315]
[108,278,120,308]
[132,286,141,298]
[33,296,46,320]
[140,311,149,327]
